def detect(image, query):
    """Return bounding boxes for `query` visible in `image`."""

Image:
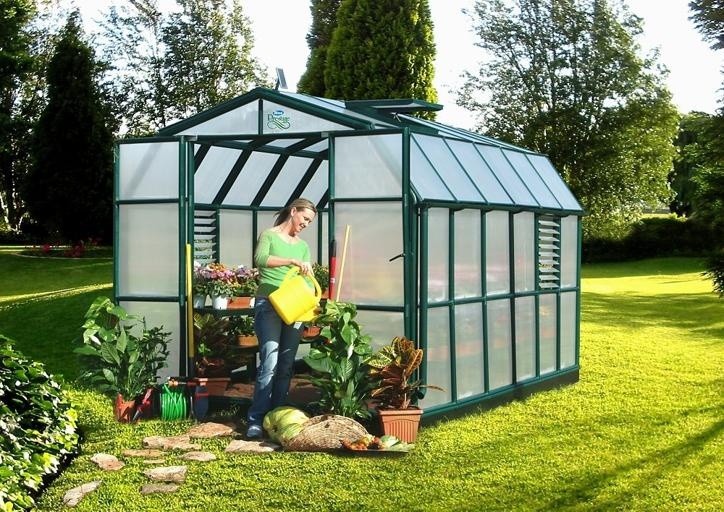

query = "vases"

[192,293,253,310]
[315,288,329,299]
[303,326,321,337]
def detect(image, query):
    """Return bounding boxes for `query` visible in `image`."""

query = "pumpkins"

[262,405,310,449]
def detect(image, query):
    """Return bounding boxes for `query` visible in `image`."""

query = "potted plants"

[72,296,172,422]
[367,337,447,444]
[192,312,235,400]
[234,317,259,347]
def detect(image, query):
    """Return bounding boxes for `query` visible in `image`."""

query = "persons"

[244,198,317,437]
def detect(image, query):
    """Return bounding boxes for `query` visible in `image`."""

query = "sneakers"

[247,423,263,439]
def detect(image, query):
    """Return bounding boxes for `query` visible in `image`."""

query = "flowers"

[312,262,330,289]
[193,261,258,296]
